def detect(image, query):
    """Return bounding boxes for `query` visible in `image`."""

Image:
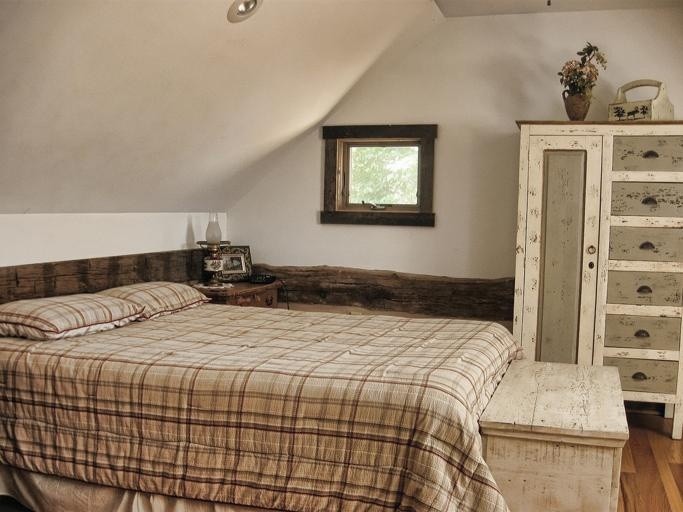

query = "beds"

[0,281,527,510]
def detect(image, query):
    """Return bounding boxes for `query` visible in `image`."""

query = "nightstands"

[191,279,288,308]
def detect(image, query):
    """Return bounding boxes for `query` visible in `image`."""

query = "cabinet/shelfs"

[510,121,603,440]
[605,125,683,439]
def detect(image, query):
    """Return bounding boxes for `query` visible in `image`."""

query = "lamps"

[196,208,232,285]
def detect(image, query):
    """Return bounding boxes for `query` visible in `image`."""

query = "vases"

[562,89,590,121]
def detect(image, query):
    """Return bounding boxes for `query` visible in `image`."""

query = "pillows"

[0,293,145,341]
[98,280,212,320]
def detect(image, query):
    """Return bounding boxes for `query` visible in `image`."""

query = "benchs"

[476,355,630,511]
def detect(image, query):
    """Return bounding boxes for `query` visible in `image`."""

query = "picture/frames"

[213,245,254,281]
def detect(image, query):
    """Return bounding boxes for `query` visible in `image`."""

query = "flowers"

[557,41,607,94]
